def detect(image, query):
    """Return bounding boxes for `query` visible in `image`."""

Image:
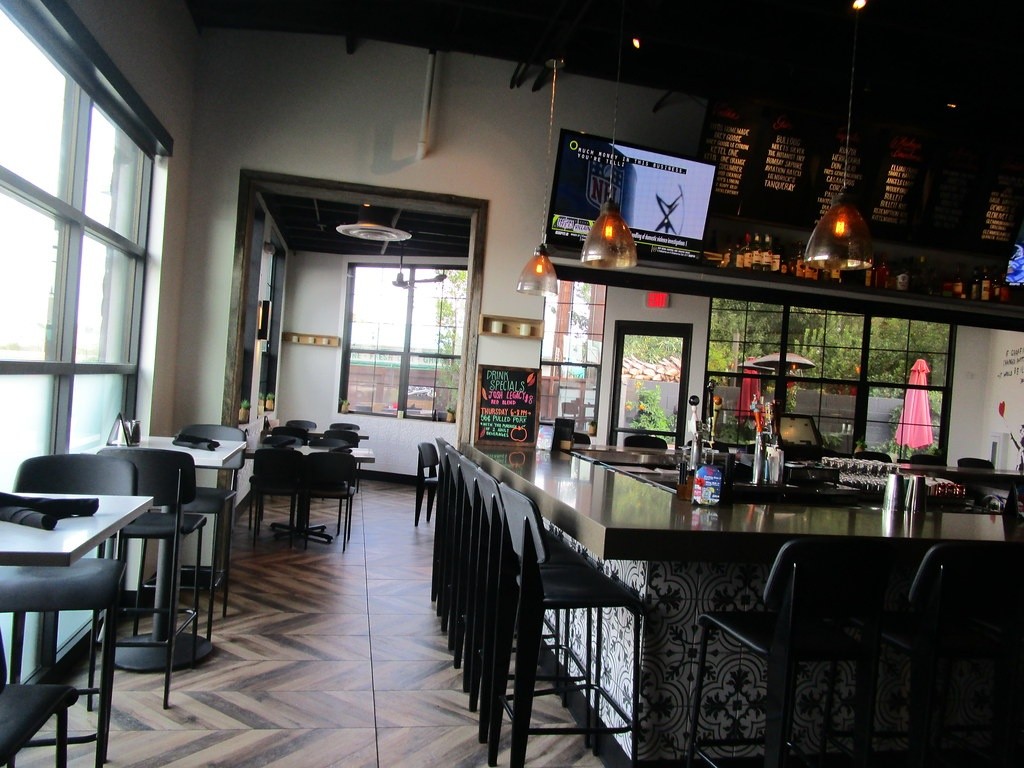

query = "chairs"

[249,419,360,551]
[414,442,439,525]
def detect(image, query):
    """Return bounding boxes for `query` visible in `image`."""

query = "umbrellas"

[892,359,934,460]
[736,355,763,433]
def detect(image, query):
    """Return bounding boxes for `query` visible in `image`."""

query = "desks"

[260,426,369,442]
[0,491,154,766]
[114,435,247,671]
[241,441,375,543]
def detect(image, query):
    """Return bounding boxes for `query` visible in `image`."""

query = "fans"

[373,229,450,292]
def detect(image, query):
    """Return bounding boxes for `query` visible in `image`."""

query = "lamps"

[516,58,559,297]
[580,0,637,269]
[804,7,875,270]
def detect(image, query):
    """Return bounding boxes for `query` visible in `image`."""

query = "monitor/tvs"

[1001,207,1024,290]
[544,127,719,267]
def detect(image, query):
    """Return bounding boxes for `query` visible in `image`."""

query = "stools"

[0,629,80,767]
[86,447,207,708]
[9,453,138,768]
[133,424,247,643]
[878,539,1023,766]
[431,434,646,768]
[683,535,892,766]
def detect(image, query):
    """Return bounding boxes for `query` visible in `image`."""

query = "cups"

[822,457,900,476]
[837,472,886,491]
[883,473,925,512]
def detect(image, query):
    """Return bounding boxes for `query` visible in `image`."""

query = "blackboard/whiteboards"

[474,364,542,448]
